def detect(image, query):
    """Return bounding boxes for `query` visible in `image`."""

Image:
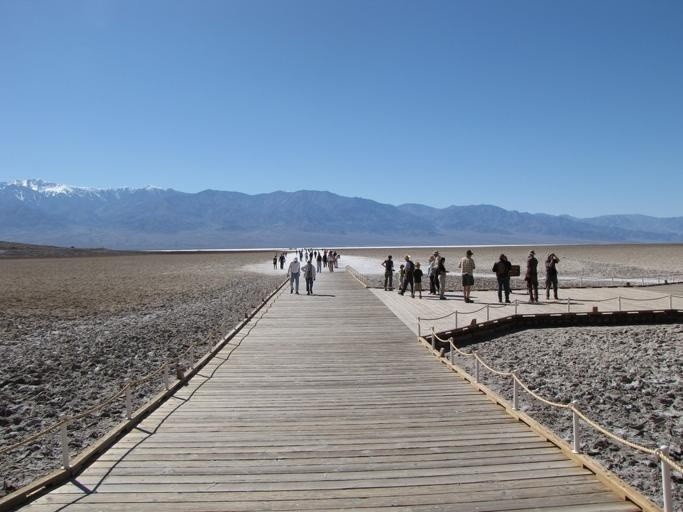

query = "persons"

[287,257,300,295]
[301,260,316,295]
[272,248,340,273]
[458,250,475,303]
[525,250,538,302]
[492,254,511,303]
[544,253,560,299]
[381,249,450,300]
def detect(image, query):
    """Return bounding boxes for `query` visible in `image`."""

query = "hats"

[466,250,473,255]
[544,252,553,261]
[528,250,536,254]
[432,250,439,255]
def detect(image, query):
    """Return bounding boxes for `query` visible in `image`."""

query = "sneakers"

[464,298,473,303]
[498,296,558,303]
[384,288,446,300]
